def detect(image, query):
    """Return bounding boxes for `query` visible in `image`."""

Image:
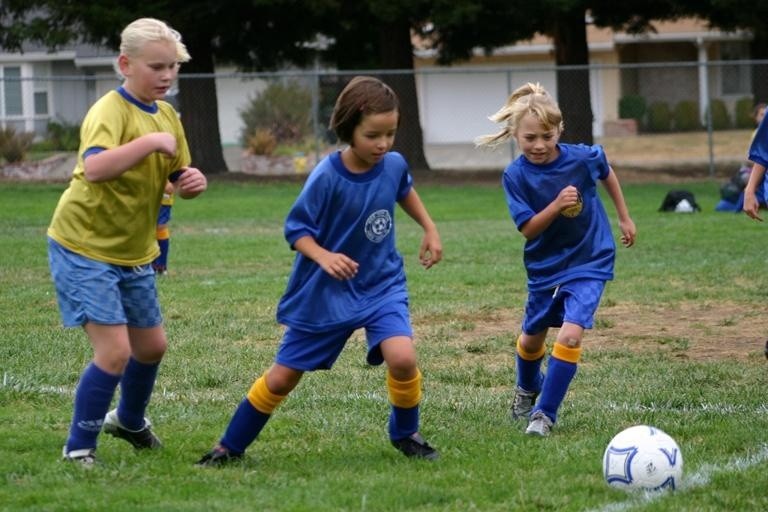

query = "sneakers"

[62,408,162,470]
[512,385,552,435]
[392,432,438,460]
[195,445,242,467]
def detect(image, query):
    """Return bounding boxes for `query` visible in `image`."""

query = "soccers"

[602,424,682,493]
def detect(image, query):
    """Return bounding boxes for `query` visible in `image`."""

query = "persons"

[470,82,638,440]
[47,16,209,475]
[187,74,444,463]
[748,102,768,148]
[742,104,767,360]
[152,173,179,277]
